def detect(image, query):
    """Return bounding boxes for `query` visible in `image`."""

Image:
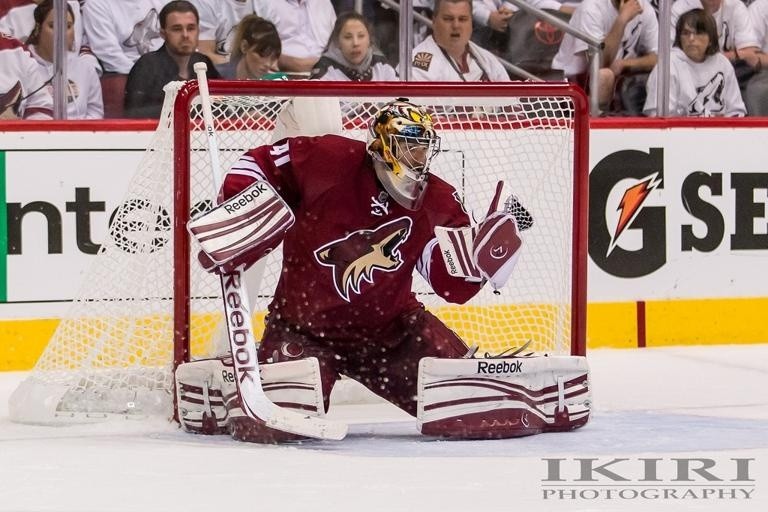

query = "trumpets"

[193,62,348,441]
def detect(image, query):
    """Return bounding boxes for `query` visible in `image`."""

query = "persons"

[0,1,766,120]
[176,98,591,446]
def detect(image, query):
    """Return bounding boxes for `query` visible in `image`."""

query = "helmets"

[366,98,441,212]
